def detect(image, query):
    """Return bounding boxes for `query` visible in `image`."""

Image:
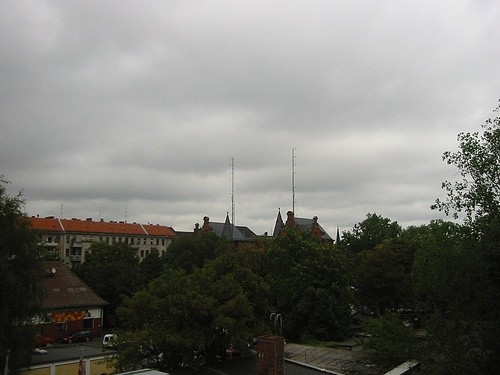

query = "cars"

[33,334,57,346]
[62,330,94,344]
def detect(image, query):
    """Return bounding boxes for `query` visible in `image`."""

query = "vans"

[103,333,120,348]
